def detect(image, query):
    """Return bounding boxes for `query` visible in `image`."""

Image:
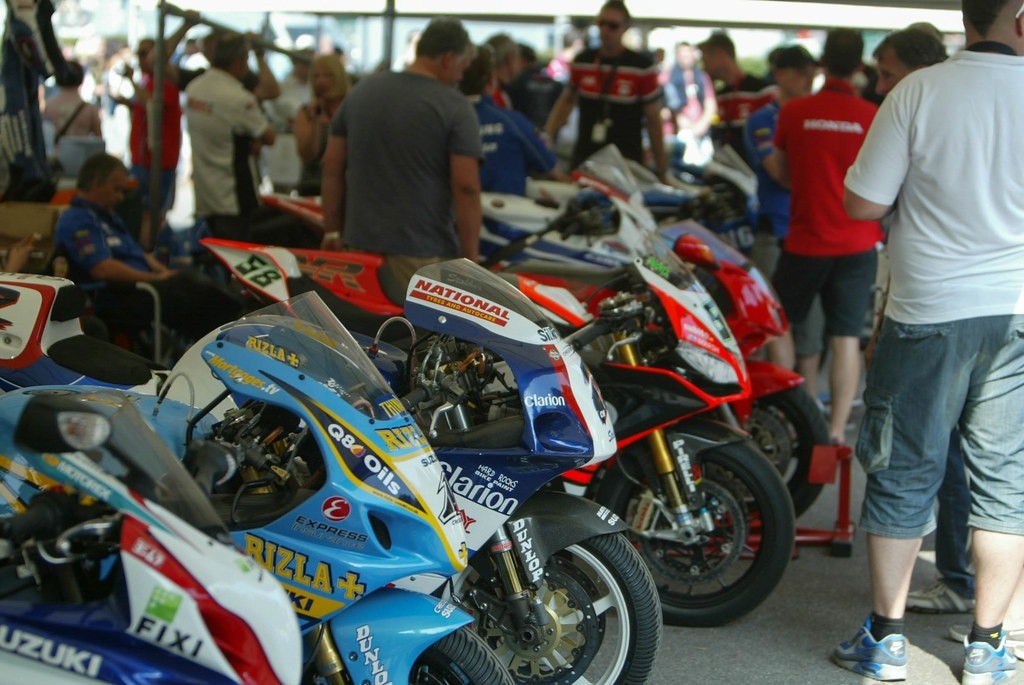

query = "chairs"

[45,241,185,368]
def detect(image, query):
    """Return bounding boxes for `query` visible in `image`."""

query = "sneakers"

[949,621,1024,661]
[961,629,1020,685]
[906,578,976,614]
[830,612,910,682]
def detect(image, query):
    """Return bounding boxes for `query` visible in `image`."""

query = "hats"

[772,44,823,71]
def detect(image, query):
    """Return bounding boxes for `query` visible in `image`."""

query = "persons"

[876,30,979,613]
[0,10,360,359]
[319,13,481,267]
[830,0,1024,685]
[459,0,1024,444]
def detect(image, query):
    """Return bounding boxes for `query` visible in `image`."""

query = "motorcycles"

[179,241,791,621]
[227,135,841,522]
[0,252,665,683]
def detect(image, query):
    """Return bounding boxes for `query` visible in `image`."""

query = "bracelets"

[323,230,341,240]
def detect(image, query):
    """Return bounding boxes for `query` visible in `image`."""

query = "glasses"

[600,18,627,29]
